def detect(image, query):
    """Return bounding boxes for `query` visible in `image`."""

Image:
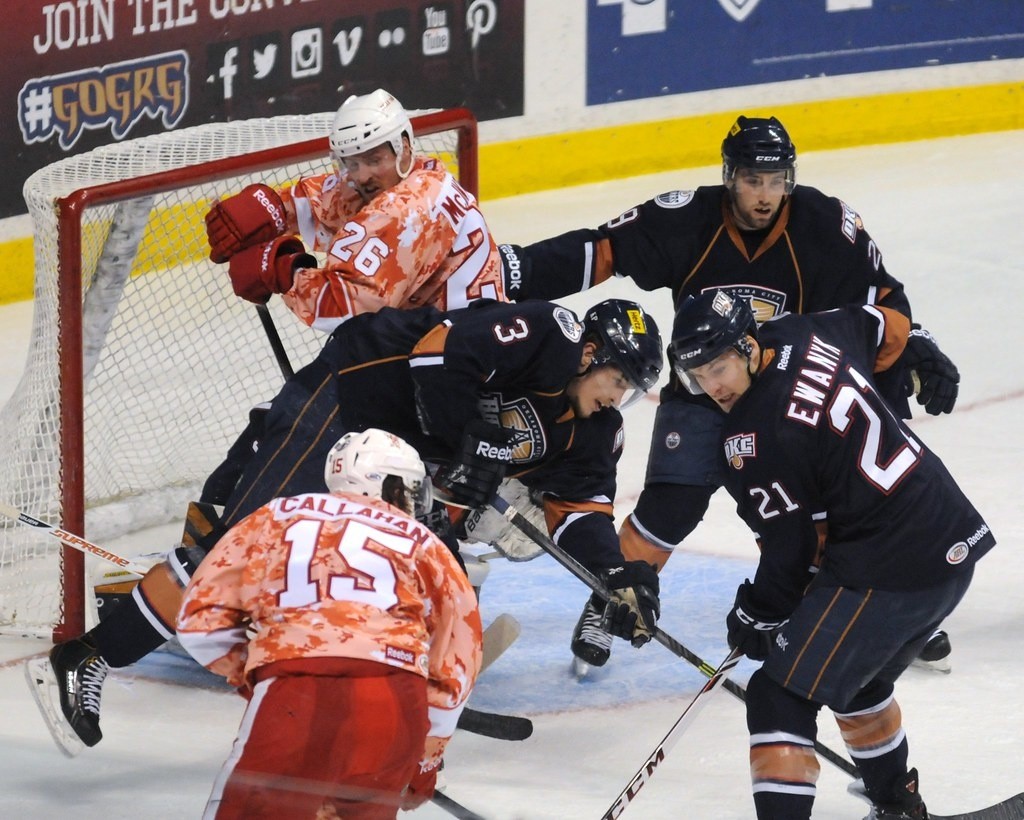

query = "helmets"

[329,88,415,170]
[324,428,425,502]
[581,299,662,394]
[668,289,760,370]
[720,114,795,194]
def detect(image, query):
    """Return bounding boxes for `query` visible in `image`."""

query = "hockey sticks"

[255,303,536,742]
[430,788,484,820]
[488,491,1024,820]
[0,504,525,673]
[601,648,743,820]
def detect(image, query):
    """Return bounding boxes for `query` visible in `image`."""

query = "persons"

[205,89,552,606]
[672,289,996,820]
[499,115,952,677]
[27,297,663,758]
[175,428,484,820]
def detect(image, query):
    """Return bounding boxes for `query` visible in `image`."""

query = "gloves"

[205,182,289,264]
[402,767,438,811]
[228,234,318,305]
[907,325,960,416]
[430,416,515,511]
[601,559,659,649]
[726,577,791,662]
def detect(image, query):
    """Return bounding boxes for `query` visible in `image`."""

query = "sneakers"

[572,590,613,677]
[907,627,953,676]
[24,631,112,756]
[855,768,926,820]
